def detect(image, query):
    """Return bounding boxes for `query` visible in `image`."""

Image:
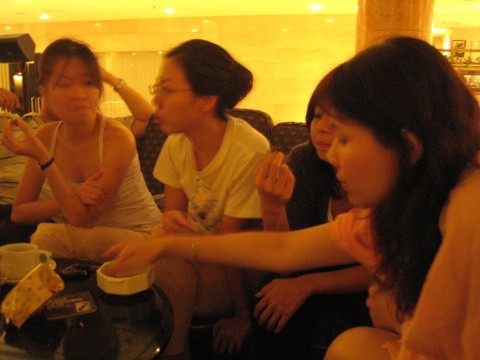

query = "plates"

[2,259,56,285]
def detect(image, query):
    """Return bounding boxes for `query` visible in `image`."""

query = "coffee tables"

[0,252,175,360]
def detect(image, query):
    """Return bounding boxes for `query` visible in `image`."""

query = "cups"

[0,244,50,279]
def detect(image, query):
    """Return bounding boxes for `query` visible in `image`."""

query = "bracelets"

[40,157,54,170]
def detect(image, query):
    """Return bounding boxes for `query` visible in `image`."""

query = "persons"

[0,37,480,360]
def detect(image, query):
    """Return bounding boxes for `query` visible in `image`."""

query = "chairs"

[130,108,315,207]
